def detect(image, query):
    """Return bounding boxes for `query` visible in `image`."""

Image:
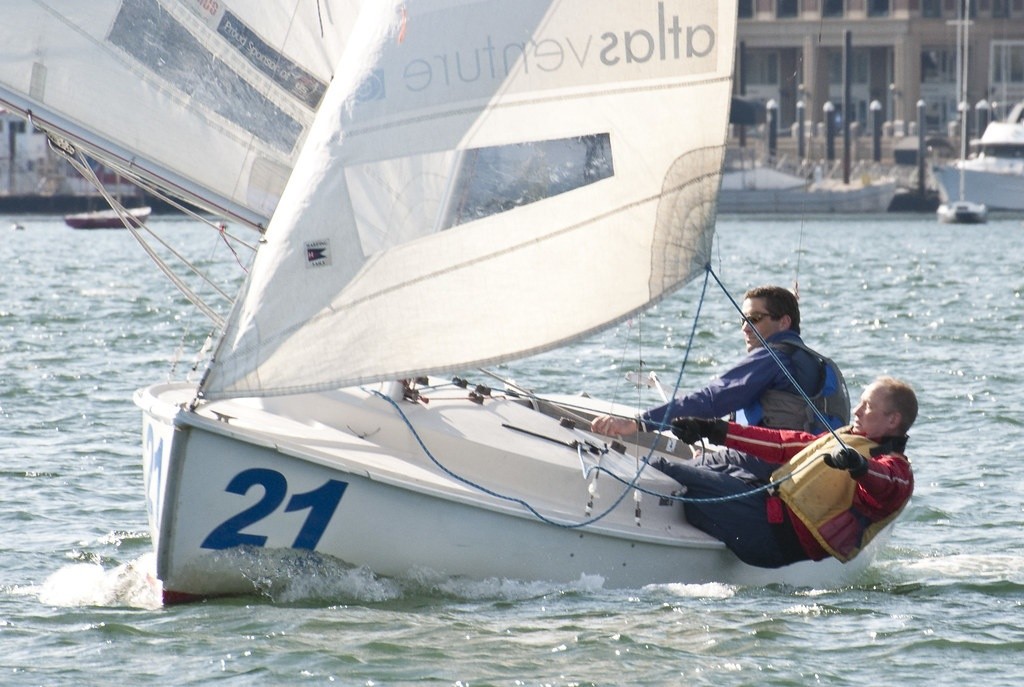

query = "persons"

[649,374,917,571]
[589,285,854,445]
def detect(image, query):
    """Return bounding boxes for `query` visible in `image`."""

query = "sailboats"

[0,0,884,612]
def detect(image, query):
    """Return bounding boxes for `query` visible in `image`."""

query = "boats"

[62,205,152,229]
[715,139,896,215]
[929,97,1024,213]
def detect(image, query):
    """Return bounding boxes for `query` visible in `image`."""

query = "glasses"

[741,311,772,326]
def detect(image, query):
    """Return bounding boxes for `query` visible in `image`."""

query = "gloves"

[672,416,726,446]
[824,443,867,478]
